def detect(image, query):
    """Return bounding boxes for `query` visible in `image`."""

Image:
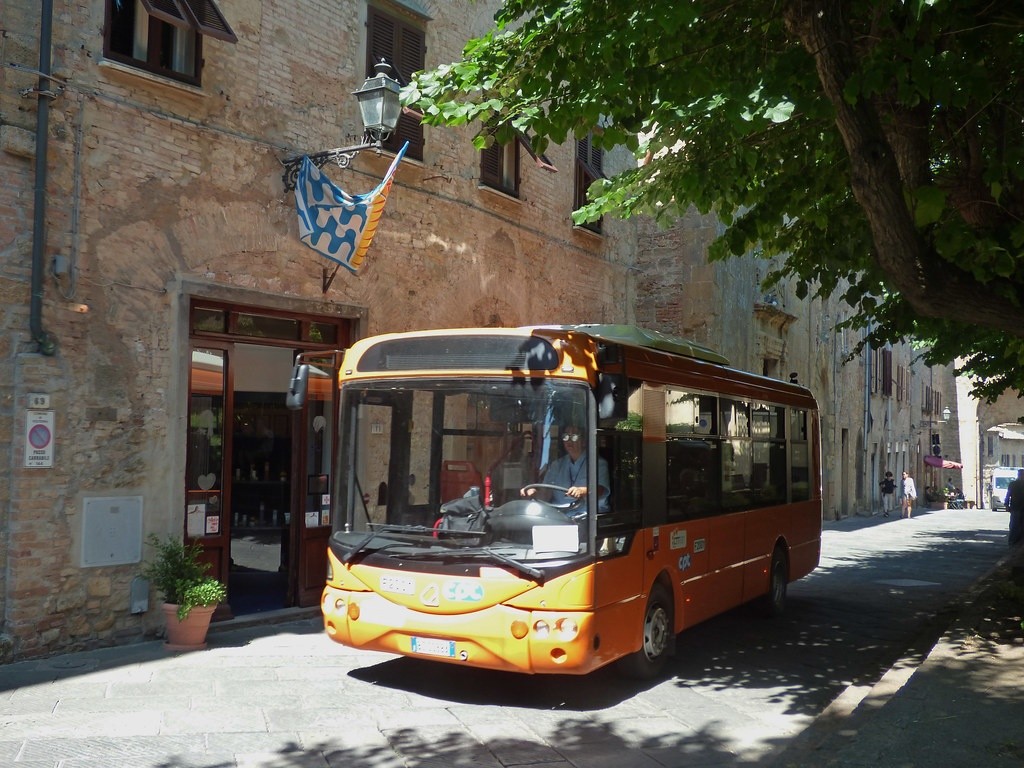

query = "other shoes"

[883,513,889,518]
[899,515,904,519]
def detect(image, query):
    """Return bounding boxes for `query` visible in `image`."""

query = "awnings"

[924,455,963,469]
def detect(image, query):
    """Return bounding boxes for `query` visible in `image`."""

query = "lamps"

[920,407,951,427]
[282,57,402,192]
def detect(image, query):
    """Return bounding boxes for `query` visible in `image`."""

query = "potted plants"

[138,532,228,646]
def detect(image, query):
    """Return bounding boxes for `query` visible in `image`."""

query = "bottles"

[471,481,480,497]
[484,475,494,510]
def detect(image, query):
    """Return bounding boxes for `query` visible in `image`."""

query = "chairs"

[440,460,482,503]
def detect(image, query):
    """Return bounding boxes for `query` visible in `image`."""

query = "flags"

[293,143,409,274]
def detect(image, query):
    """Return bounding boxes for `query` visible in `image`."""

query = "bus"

[285,323,823,680]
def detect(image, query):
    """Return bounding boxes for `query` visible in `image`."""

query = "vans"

[987,467,1024,512]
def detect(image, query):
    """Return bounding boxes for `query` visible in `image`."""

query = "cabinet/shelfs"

[232,481,290,531]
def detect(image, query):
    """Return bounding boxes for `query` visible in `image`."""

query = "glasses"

[887,476,890,477]
[562,433,583,441]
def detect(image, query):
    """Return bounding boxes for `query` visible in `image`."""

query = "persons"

[1004,469,1024,542]
[900,471,917,518]
[879,471,898,518]
[522,420,613,517]
[946,478,956,492]
[926,486,930,494]
